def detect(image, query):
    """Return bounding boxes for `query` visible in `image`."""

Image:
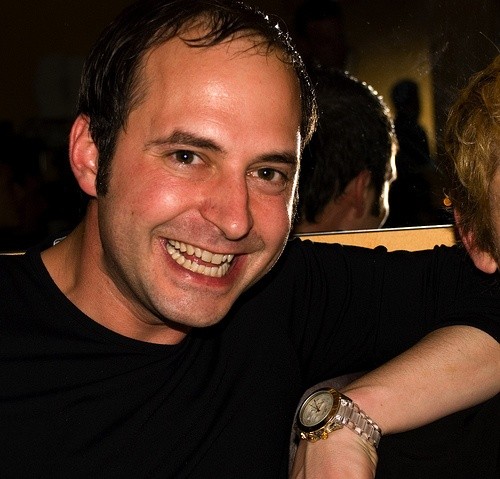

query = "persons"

[432,52,500,289]
[427,32,491,129]
[0,0,499,479]
[295,61,397,234]
[388,79,439,229]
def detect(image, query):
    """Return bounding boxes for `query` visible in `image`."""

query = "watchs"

[293,386,382,449]
